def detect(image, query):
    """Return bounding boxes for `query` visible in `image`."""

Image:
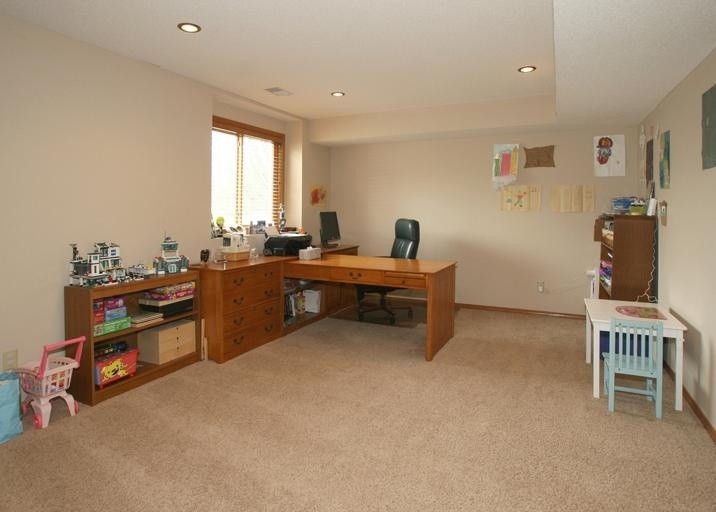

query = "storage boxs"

[601,331,667,361]
[138,320,197,365]
[95,349,139,390]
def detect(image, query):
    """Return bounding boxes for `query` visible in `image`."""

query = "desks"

[584,298,687,412]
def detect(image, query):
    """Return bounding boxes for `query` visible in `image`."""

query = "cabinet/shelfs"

[188,244,457,364]
[592,215,658,302]
[64,270,202,407]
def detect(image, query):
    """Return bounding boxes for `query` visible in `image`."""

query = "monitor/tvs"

[319,212,340,248]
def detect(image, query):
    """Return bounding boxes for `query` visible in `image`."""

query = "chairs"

[355,218,419,324]
[602,318,663,419]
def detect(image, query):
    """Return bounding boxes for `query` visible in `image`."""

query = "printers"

[265,231,312,256]
